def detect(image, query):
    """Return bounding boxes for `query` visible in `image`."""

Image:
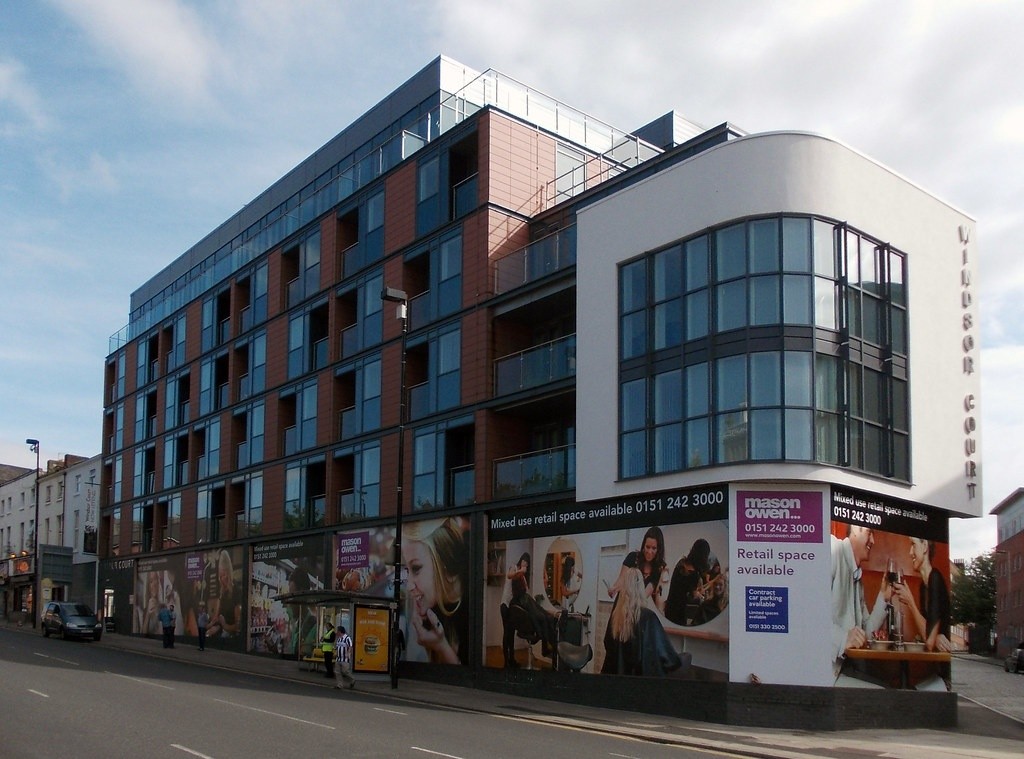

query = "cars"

[1003,642,1024,674]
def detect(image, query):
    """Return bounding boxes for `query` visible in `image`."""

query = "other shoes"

[337,685,343,689]
[198,647,204,651]
[505,659,510,668]
[511,660,521,668]
[350,679,356,688]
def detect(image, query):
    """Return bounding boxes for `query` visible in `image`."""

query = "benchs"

[303,649,336,673]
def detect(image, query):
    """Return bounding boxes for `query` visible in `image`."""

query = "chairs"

[509,604,544,672]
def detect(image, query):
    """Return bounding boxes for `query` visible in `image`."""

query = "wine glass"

[886,558,898,607]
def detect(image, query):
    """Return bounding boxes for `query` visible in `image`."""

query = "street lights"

[379,284,409,688]
[25,438,41,628]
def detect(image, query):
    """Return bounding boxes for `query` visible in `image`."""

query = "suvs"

[40,601,103,642]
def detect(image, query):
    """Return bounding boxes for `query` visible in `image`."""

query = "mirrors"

[655,521,729,625]
[541,536,584,610]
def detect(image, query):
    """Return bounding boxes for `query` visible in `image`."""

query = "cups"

[895,568,905,585]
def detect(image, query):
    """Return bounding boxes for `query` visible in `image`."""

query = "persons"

[133,561,186,637]
[196,605,209,651]
[401,516,472,665]
[828,522,898,691]
[265,567,318,662]
[158,604,176,649]
[207,550,242,640]
[892,535,951,692]
[320,622,337,679]
[334,625,356,691]
[598,526,729,680]
[497,551,560,673]
[557,556,583,611]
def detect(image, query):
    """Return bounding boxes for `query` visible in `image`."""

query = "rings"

[434,621,442,628]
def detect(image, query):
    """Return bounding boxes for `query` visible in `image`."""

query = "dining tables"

[843,641,951,690]
